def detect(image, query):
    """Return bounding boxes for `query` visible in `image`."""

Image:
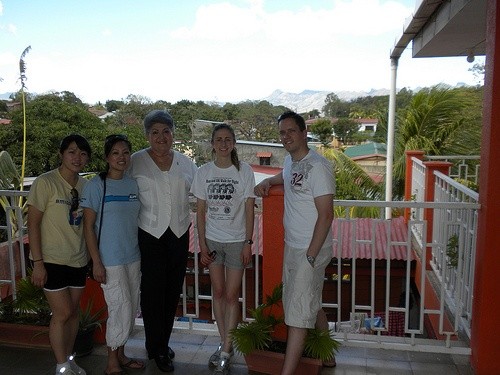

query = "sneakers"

[55,361,76,375]
[66,352,86,375]
[208,342,224,367]
[215,351,234,372]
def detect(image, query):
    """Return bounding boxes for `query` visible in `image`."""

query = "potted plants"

[227,282,342,375]
[0,267,54,352]
[31,296,108,358]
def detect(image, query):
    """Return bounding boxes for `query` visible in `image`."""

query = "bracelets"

[32,259,44,262]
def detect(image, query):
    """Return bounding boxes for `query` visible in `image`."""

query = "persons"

[25,134,92,375]
[253,111,334,375]
[82,136,146,375]
[126,110,198,374]
[190,123,257,375]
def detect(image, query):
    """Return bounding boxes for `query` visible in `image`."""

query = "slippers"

[104,370,127,375]
[121,358,145,370]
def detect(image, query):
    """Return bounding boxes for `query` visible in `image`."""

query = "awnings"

[185,212,417,262]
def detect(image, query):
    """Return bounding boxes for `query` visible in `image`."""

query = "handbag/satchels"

[86,258,93,278]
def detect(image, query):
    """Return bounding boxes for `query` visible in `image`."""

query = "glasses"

[105,134,128,145]
[209,251,217,260]
[71,188,79,209]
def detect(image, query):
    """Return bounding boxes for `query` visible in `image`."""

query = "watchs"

[307,255,315,263]
[245,239,253,244]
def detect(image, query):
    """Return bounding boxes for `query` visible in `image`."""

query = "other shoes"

[152,349,174,372]
[146,345,175,359]
[323,351,336,367]
